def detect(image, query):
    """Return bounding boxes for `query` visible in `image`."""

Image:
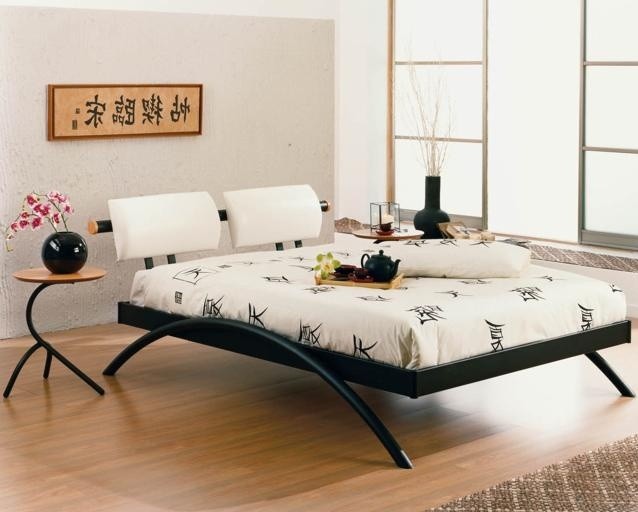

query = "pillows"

[375,238,533,280]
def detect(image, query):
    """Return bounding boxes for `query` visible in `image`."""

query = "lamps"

[368,201,403,233]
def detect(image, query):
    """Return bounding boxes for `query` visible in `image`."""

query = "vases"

[38,230,88,276]
[411,176,451,240]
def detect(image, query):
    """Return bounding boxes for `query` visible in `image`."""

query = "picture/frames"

[44,81,204,145]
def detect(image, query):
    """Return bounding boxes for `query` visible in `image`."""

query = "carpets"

[330,216,635,272]
[426,431,637,510]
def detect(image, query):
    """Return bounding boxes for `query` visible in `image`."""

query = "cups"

[380,224,393,231]
[334,265,368,278]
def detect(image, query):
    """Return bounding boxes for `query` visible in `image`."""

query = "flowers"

[304,248,340,281]
[0,182,75,253]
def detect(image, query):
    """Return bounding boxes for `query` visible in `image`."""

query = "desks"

[1,262,107,402]
[350,226,426,243]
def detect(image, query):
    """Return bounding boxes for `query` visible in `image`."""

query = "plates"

[376,231,397,236]
[329,273,377,283]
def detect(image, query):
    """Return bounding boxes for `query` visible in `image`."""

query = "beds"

[84,182,635,471]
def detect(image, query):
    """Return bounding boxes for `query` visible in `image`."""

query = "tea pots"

[361,247,400,282]
